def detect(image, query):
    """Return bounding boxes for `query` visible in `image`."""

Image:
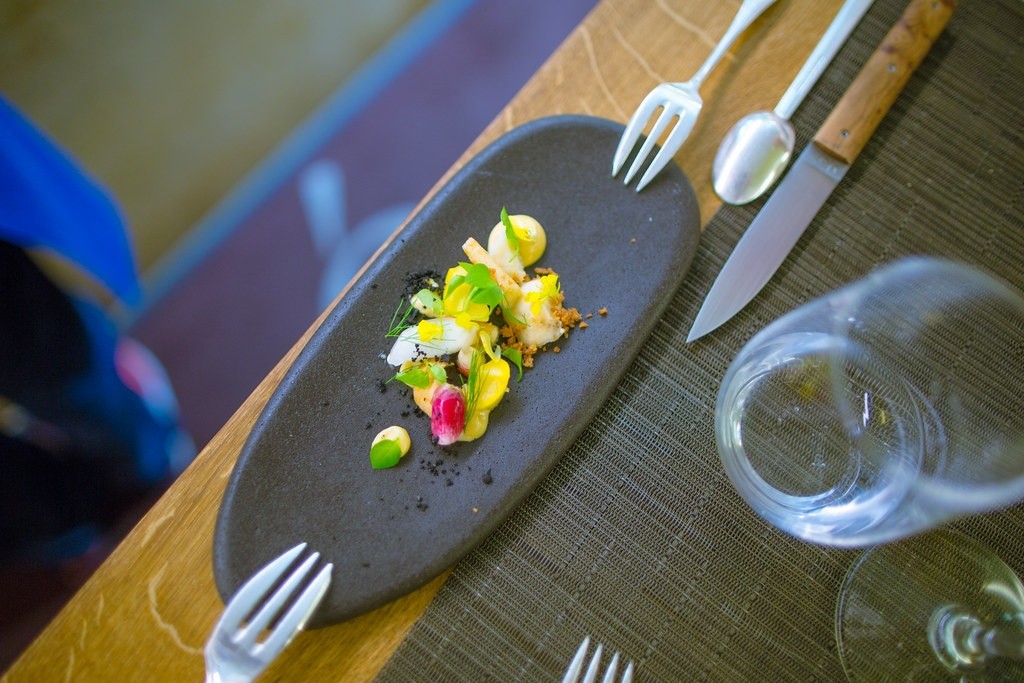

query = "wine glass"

[835,528,1024,683]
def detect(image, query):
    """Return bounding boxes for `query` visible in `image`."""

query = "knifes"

[684,0,958,344]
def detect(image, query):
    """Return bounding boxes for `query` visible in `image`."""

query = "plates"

[211,116,701,630]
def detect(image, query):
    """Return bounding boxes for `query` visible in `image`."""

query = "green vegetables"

[384,262,531,391]
[369,439,401,469]
[500,207,518,263]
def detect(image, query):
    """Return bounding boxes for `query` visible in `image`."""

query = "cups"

[713,256,1024,547]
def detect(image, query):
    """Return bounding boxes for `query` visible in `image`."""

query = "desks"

[0,0,1024,683]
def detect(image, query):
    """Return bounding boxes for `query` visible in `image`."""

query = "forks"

[202,542,335,683]
[612,0,778,192]
[560,636,635,683]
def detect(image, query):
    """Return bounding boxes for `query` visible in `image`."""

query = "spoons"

[711,0,874,205]
